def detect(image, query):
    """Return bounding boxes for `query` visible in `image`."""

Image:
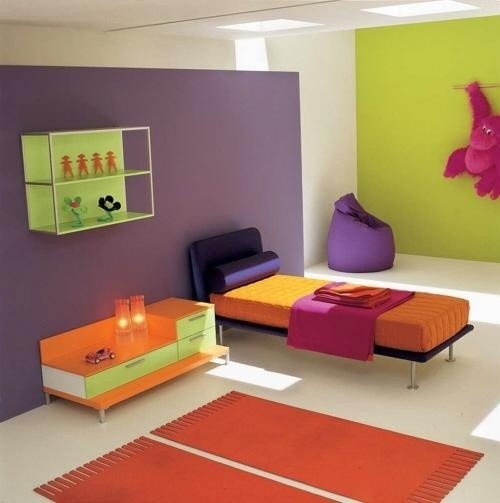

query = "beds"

[189,226,473,391]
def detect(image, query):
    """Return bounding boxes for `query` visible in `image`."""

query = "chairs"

[328,202,395,273]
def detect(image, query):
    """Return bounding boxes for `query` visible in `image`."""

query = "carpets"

[34,436,341,503]
[149,390,485,503]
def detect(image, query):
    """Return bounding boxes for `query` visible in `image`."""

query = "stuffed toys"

[62,195,87,223]
[442,80,499,199]
[96,195,121,221]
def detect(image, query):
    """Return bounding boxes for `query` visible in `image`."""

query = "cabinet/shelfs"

[17,125,155,236]
[39,297,230,424]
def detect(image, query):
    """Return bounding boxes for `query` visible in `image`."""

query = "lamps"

[130,296,147,332]
[115,299,130,335]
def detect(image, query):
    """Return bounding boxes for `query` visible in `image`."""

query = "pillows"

[211,251,281,293]
[334,192,370,225]
